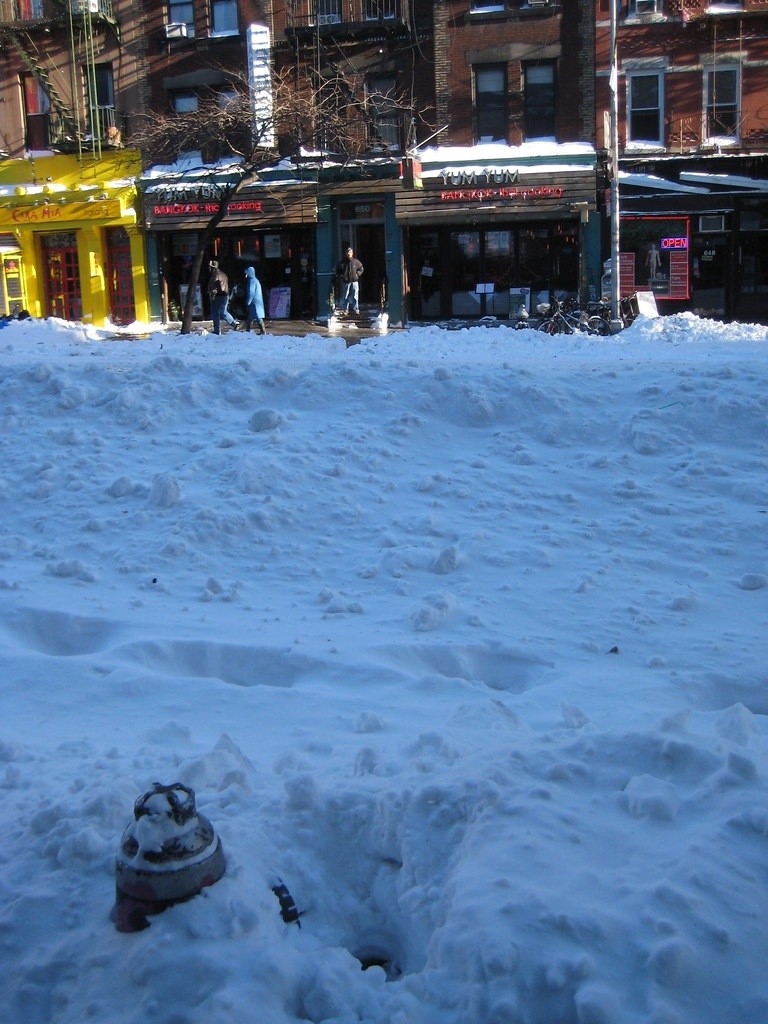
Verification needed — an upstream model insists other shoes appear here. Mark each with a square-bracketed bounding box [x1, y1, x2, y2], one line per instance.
[234, 324, 240, 330]
[257, 331, 265, 335]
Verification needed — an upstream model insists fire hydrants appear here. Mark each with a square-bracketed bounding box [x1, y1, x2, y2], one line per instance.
[112, 780, 300, 937]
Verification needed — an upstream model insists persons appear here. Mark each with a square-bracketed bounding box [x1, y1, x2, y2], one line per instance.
[335, 247, 364, 316]
[244, 267, 268, 335]
[645, 244, 662, 279]
[209, 260, 241, 336]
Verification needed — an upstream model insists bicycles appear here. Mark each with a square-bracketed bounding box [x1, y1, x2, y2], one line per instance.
[535, 290, 637, 338]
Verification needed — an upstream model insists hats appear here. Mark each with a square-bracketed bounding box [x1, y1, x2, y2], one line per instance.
[209, 260, 218, 268]
